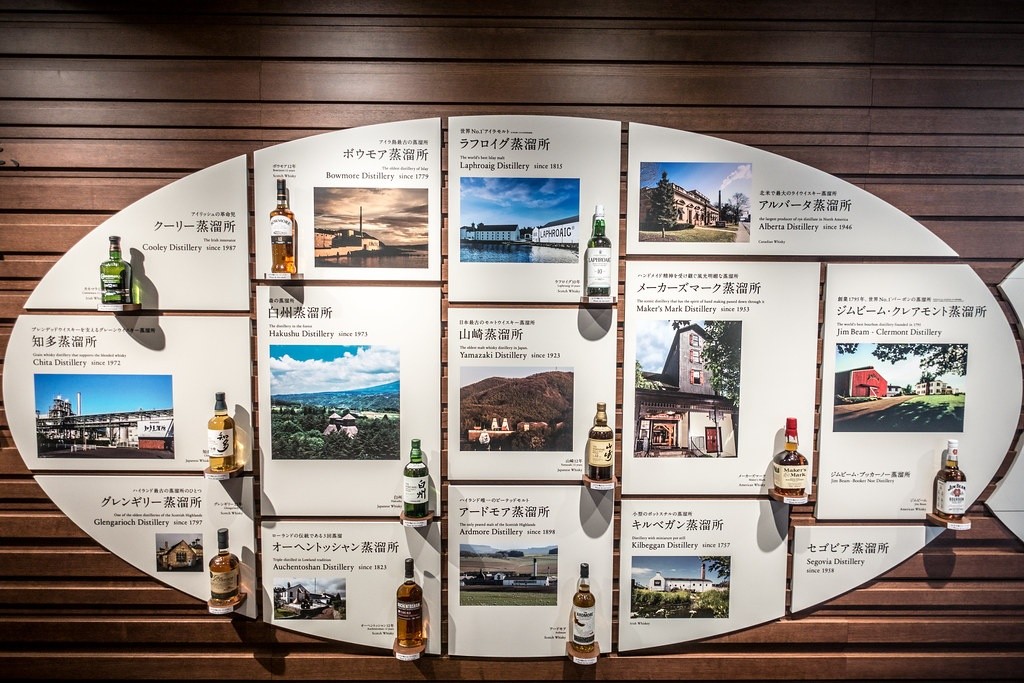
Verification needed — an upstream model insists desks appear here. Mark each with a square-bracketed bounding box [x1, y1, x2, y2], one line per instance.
[469, 430, 516, 451]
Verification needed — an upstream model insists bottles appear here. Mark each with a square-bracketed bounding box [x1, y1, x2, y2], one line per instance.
[403, 438, 429, 518]
[584, 206, 612, 297]
[772, 418, 808, 497]
[587, 402, 613, 481]
[207, 392, 236, 473]
[572, 562, 595, 652]
[98, 235, 134, 304]
[397, 558, 423, 648]
[208, 529, 241, 606]
[269, 178, 298, 274]
[935, 440, 967, 522]
